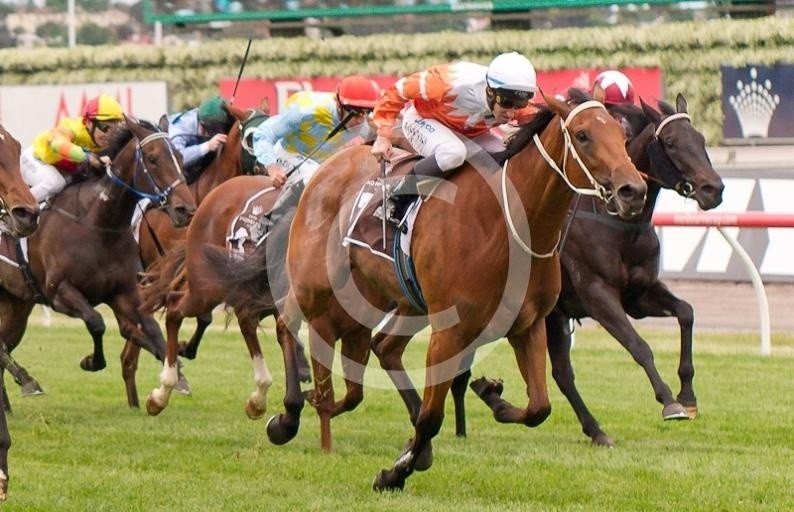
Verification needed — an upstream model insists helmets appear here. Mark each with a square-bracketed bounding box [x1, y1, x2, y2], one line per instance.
[486, 51, 537, 93]
[197, 97, 230, 131]
[592, 71, 634, 106]
[81, 95, 125, 126]
[336, 76, 381, 108]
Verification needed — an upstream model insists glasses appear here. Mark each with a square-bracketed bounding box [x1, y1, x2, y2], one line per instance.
[344, 108, 370, 118]
[493, 93, 528, 109]
[96, 123, 111, 133]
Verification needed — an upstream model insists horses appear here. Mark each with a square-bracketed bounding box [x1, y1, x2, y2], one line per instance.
[0, 80, 726, 501]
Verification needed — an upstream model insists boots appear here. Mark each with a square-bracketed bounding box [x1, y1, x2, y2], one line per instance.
[372, 167, 421, 235]
[255, 186, 297, 242]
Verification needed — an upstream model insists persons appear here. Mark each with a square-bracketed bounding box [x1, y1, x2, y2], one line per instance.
[20, 95, 124, 203]
[249, 76, 384, 245]
[554, 69, 635, 143]
[372, 50, 537, 235]
[150, 96, 232, 166]
